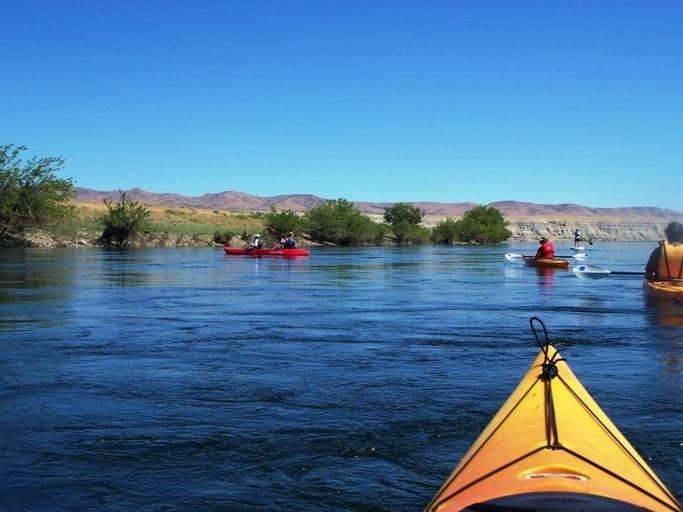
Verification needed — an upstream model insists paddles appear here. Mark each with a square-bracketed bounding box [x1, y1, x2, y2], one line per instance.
[505, 253, 589, 261]
[572, 265, 647, 275]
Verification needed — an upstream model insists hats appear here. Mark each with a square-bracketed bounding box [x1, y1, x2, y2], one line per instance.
[255, 234, 260, 237]
[538, 237, 548, 242]
[286, 232, 294, 236]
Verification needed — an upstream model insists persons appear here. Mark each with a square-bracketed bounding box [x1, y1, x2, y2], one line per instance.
[532, 235, 555, 260]
[643, 221, 682, 280]
[571, 228, 581, 247]
[283, 231, 296, 249]
[247, 233, 263, 249]
[270, 234, 284, 248]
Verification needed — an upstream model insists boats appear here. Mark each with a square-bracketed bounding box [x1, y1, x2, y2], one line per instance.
[422, 317, 683, 512]
[569, 247, 585, 250]
[524, 257, 571, 269]
[641, 276, 683, 303]
[223, 246, 310, 256]
